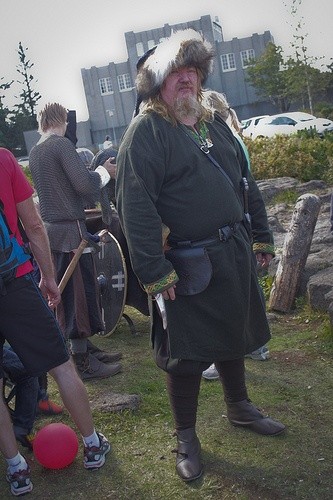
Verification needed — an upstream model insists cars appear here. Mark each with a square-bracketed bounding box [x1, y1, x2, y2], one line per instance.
[251, 112, 333, 142]
[241, 115, 272, 138]
[75, 146, 95, 168]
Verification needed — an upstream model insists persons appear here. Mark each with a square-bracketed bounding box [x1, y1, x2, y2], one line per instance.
[0, 147, 111, 496]
[3, 343, 63, 452]
[114, 28, 285, 481]
[202, 91, 269, 380]
[29, 102, 124, 381]
[103, 135, 113, 149]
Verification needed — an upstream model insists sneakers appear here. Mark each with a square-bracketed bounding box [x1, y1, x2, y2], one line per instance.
[83, 432, 109, 469]
[5, 464, 32, 495]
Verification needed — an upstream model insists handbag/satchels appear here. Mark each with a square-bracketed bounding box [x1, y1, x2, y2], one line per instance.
[163, 248, 212, 296]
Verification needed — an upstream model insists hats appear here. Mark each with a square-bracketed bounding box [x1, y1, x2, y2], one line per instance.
[134, 28, 214, 97]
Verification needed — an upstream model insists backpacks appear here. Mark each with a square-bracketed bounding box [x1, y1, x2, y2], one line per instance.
[0, 198, 33, 286]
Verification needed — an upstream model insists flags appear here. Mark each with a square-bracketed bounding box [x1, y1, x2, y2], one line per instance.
[33, 423, 79, 469]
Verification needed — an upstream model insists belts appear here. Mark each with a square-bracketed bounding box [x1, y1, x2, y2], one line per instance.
[168, 222, 241, 248]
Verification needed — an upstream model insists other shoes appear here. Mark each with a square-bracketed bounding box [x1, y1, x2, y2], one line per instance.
[69, 352, 121, 380]
[86, 342, 121, 363]
[36, 393, 61, 413]
[174, 428, 203, 481]
[202, 362, 219, 379]
[225, 400, 286, 436]
[244, 343, 269, 360]
